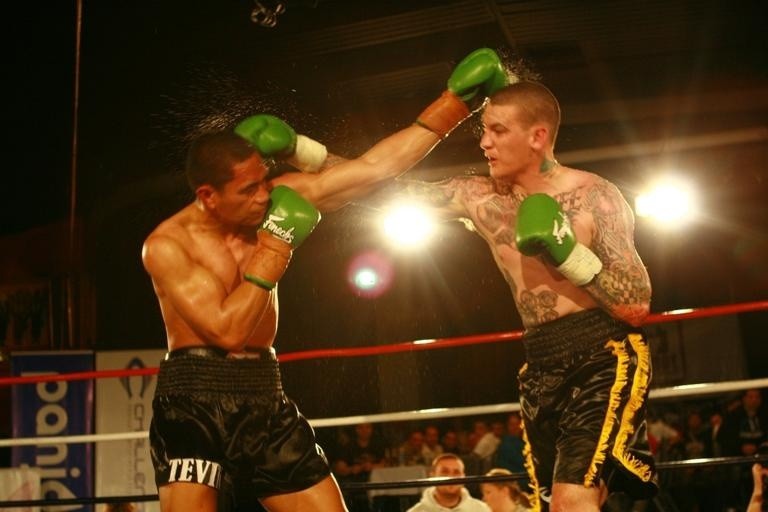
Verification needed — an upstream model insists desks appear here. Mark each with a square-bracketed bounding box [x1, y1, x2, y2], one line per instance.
[369, 465, 427, 512]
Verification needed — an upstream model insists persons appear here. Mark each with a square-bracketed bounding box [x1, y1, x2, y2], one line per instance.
[141, 47, 507, 512]
[325, 389, 767, 512]
[235, 81, 660, 512]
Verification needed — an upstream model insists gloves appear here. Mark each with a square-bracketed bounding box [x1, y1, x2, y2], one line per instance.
[516, 193, 603, 286]
[416, 47, 508, 139]
[243, 185, 322, 290]
[234, 115, 328, 174]
